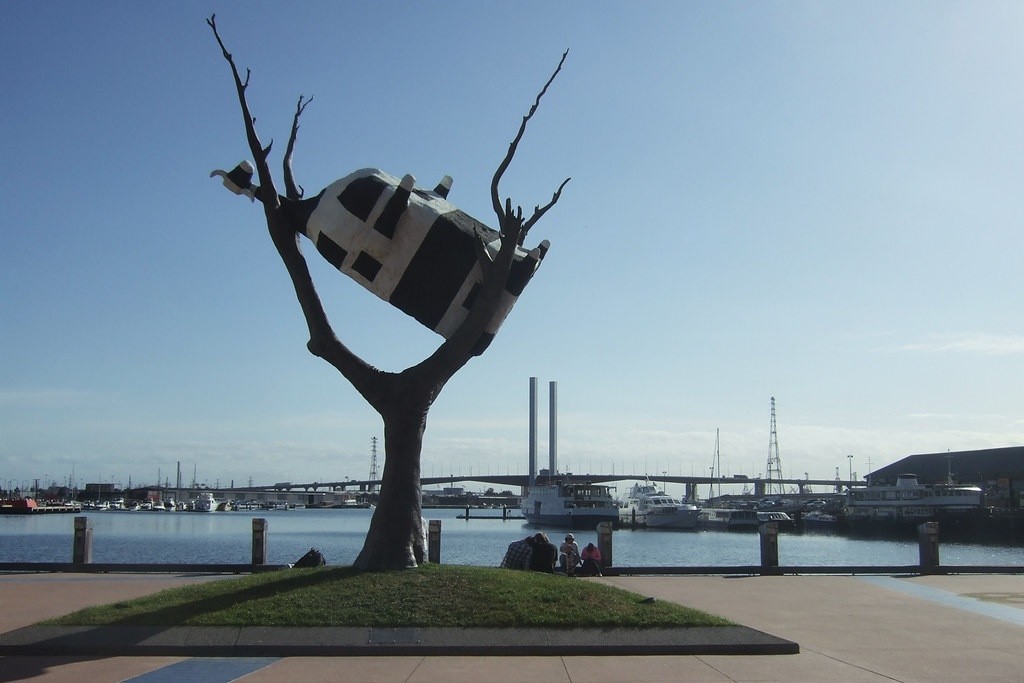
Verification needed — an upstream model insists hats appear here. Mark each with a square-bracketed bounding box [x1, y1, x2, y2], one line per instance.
[567, 533, 574, 539]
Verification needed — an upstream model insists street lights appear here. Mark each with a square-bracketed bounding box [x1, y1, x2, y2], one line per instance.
[847, 455, 853, 487]
[662, 471, 666, 493]
[8, 478, 17, 491]
[0, 478, 6, 489]
[709, 467, 714, 490]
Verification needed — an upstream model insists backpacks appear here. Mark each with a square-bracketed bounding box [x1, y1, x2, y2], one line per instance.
[581, 559, 600, 574]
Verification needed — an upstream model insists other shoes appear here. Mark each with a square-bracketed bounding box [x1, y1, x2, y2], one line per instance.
[598, 571, 603, 577]
[573, 570, 577, 576]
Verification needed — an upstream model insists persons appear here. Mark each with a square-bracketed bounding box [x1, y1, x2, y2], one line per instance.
[499, 533, 558, 573]
[560, 534, 580, 577]
[582, 543, 604, 577]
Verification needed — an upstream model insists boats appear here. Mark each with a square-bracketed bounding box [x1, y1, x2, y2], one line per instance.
[520, 469, 1024, 531]
[38, 493, 240, 512]
